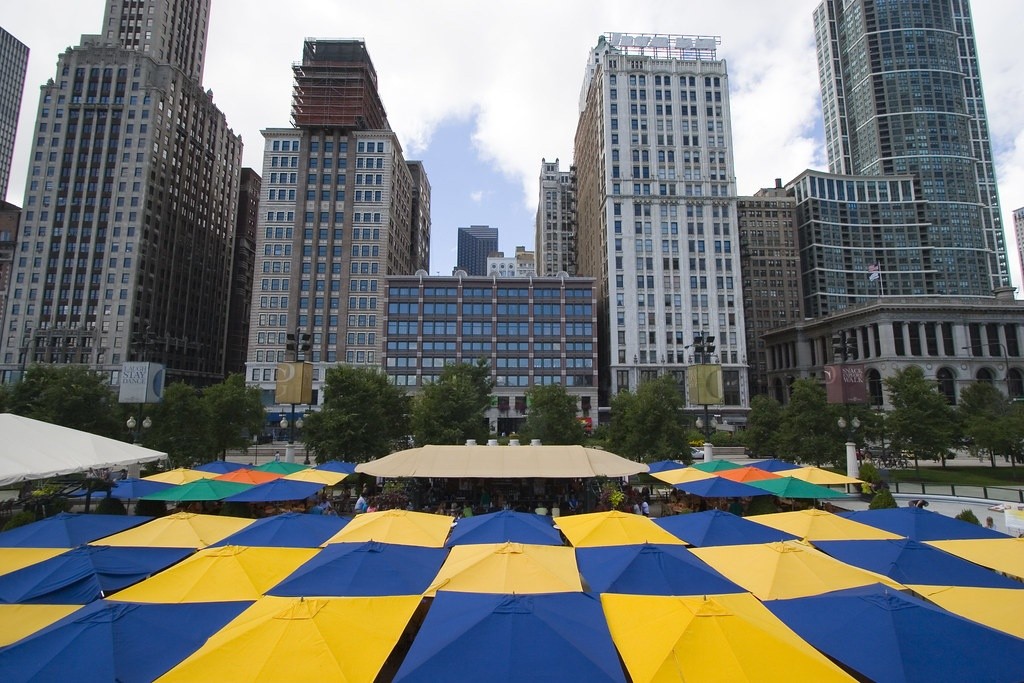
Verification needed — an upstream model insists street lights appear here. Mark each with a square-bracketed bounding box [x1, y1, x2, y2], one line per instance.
[116, 326, 166, 514]
[824, 329, 869, 492]
[685, 331, 725, 462]
[963, 342, 1011, 402]
[272, 326, 313, 466]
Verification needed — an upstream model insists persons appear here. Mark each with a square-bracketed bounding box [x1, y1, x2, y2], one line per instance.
[909, 499, 929, 510]
[310, 480, 840, 544]
[984, 516, 996, 531]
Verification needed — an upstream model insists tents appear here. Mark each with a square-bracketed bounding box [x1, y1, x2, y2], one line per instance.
[0, 413, 167, 484]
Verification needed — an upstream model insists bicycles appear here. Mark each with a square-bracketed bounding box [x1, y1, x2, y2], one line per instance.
[868, 457, 914, 469]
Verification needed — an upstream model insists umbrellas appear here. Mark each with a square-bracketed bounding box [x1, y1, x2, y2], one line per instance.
[646, 459, 867, 498]
[1, 509, 1024, 683]
[108, 459, 357, 503]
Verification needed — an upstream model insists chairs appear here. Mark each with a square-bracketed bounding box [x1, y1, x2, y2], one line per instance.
[0, 497, 16, 517]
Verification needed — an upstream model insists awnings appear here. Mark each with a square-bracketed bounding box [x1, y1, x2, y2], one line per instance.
[354, 444, 649, 478]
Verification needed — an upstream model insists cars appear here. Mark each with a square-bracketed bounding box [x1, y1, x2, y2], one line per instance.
[688, 447, 705, 459]
[388, 435, 417, 450]
[856, 445, 918, 461]
